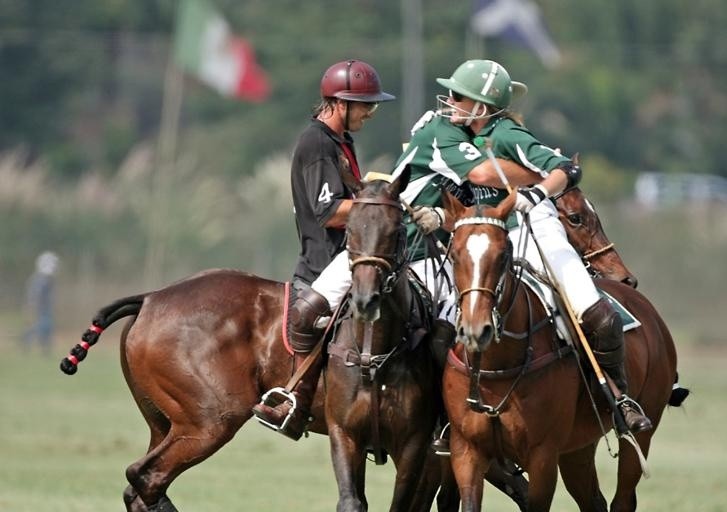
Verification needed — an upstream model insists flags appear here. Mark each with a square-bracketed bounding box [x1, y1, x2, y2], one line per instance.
[468, 0, 562, 71]
[165, 0, 271, 102]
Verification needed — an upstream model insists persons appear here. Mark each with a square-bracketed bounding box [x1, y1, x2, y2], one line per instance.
[14, 250, 61, 357]
[251, 80, 546, 441]
[286, 57, 396, 286]
[409, 59, 654, 437]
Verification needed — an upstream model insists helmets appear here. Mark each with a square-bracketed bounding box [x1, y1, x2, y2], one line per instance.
[435, 59, 514, 110]
[317, 58, 397, 103]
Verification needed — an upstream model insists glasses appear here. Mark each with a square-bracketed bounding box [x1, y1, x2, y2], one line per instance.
[447, 87, 467, 103]
[355, 100, 379, 113]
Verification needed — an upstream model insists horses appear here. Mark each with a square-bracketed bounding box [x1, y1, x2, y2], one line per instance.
[59, 148, 689, 511]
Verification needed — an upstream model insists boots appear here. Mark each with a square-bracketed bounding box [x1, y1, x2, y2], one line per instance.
[430, 425, 452, 452]
[253, 351, 324, 441]
[583, 298, 654, 434]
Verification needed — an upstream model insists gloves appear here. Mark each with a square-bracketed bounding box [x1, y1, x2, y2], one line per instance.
[409, 108, 435, 137]
[510, 183, 547, 215]
[407, 204, 446, 236]
